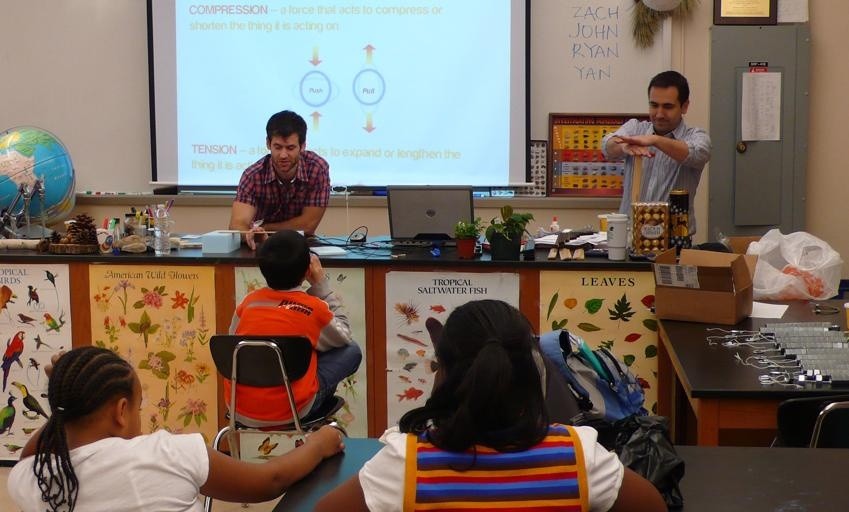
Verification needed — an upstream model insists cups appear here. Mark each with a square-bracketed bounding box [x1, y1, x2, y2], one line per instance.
[608, 215, 628, 261]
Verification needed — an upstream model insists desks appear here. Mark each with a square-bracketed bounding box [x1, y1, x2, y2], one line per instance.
[653, 296, 849, 448]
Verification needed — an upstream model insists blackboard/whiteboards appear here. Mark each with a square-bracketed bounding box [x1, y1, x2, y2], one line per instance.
[0, 1, 686, 210]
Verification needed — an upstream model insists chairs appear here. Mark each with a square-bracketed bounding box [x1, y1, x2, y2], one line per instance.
[770, 394, 849, 450]
[200, 334, 351, 511]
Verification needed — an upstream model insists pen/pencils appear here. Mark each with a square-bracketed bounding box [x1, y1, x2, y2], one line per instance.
[87, 190, 118, 196]
[147, 198, 174, 217]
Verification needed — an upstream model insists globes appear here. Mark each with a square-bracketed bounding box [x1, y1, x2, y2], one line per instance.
[0, 124, 75, 238]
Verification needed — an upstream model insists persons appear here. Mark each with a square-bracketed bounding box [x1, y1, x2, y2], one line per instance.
[601, 71, 713, 248]
[224, 229, 362, 428]
[229, 109, 331, 251]
[7, 345, 346, 512]
[311, 299, 669, 512]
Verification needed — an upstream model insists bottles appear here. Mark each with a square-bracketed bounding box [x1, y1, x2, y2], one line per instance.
[122, 214, 140, 238]
[550, 216, 560, 235]
[156, 204, 170, 255]
[670, 190, 689, 254]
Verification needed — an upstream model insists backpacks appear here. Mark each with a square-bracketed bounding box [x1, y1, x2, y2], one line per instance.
[536, 329, 649, 455]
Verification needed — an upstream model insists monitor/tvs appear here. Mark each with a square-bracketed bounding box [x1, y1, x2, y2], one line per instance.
[388, 183, 474, 249]
